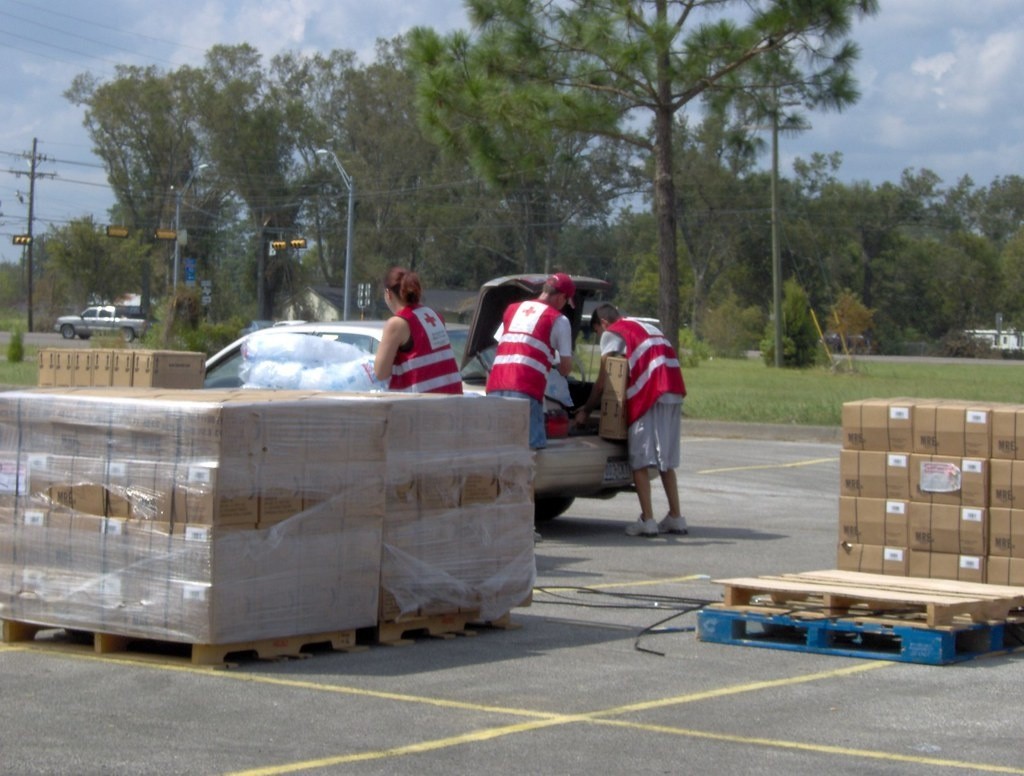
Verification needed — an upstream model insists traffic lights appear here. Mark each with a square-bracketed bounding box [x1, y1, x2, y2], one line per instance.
[272, 241, 286, 249]
[290, 239, 307, 248]
[13, 235, 32, 244]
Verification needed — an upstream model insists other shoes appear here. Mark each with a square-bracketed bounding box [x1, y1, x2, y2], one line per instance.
[533, 528, 542, 542]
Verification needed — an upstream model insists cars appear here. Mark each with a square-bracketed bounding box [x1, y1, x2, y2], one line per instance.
[201, 274, 661, 521]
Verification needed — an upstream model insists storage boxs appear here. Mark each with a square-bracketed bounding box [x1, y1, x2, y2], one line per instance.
[597, 356, 631, 439]
[836, 397, 1023, 587]
[0, 348, 538, 648]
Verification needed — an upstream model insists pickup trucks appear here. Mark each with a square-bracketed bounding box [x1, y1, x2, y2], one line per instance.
[53, 305, 145, 342]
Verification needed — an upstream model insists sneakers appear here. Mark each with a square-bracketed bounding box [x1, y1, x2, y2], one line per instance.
[658, 513, 688, 533]
[625, 513, 659, 536]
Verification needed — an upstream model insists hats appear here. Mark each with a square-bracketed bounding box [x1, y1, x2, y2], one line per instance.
[546, 273, 576, 309]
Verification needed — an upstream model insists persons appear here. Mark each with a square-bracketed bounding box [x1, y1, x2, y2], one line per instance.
[482, 274, 575, 544]
[373, 265, 465, 395]
[574, 304, 690, 537]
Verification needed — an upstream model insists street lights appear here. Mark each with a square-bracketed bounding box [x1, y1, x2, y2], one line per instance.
[173, 164, 208, 293]
[316, 148, 355, 322]
